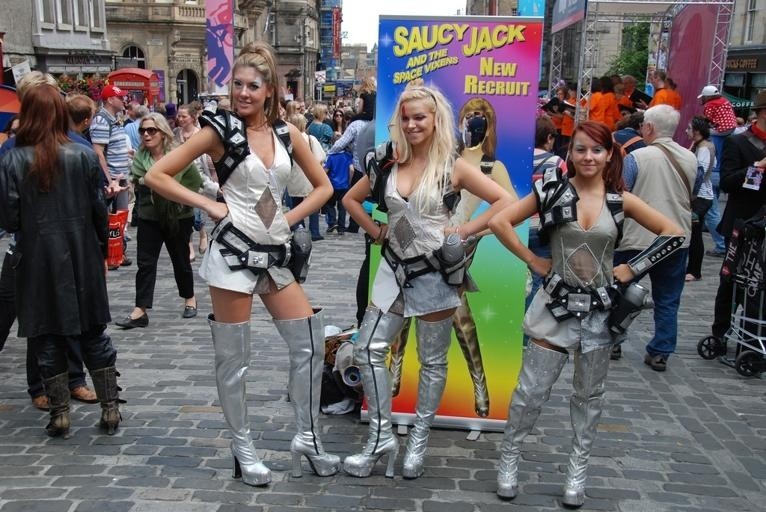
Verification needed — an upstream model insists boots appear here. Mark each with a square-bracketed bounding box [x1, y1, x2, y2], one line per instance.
[390, 316, 412, 397]
[453, 293, 489, 417]
[89, 366, 124, 434]
[44, 371, 70, 439]
[272, 306, 341, 478]
[343, 307, 400, 477]
[498, 337, 569, 498]
[402, 314, 455, 477]
[563, 344, 614, 506]
[208, 313, 270, 486]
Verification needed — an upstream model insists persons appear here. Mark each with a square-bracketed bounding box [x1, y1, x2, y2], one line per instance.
[145, 42, 342, 483]
[488, 122, 685, 502]
[2, 42, 765, 438]
[341, 83, 514, 478]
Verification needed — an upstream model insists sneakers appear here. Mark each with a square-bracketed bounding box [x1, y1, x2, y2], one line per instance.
[311, 222, 357, 241]
[199, 232, 208, 255]
[611, 345, 621, 359]
[645, 353, 666, 371]
[190, 256, 196, 262]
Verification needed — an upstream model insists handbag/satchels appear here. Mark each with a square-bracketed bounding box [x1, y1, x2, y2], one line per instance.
[103, 196, 129, 267]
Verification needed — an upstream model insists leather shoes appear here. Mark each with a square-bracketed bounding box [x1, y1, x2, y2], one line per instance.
[70, 386, 99, 403]
[183, 301, 197, 318]
[31, 396, 50, 410]
[115, 313, 148, 328]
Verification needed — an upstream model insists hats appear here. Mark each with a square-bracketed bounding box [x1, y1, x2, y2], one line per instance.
[750, 89, 766, 109]
[101, 86, 129, 99]
[697, 85, 720, 100]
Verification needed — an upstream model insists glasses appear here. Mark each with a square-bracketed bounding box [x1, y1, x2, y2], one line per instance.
[138, 127, 161, 135]
